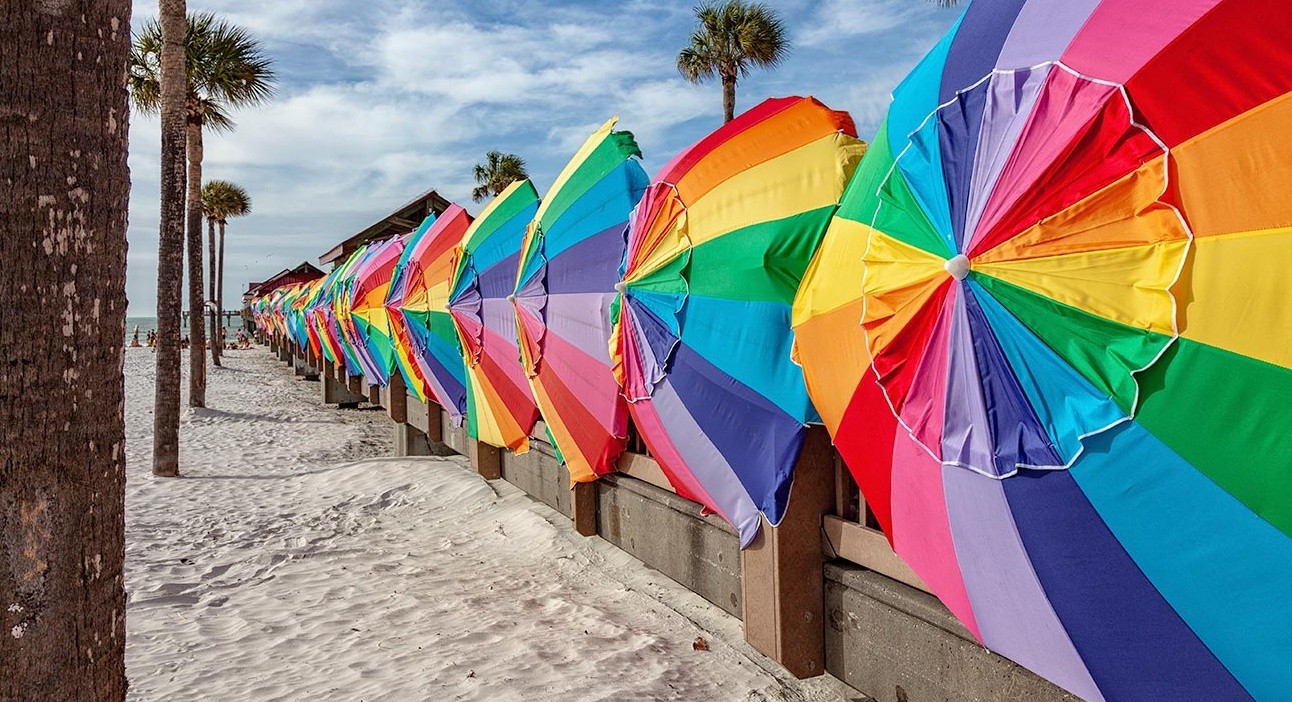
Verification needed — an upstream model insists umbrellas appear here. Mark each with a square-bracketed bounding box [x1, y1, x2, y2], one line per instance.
[506, 119, 652, 492]
[790, 0, 1292, 702]
[445, 176, 543, 456]
[381, 211, 438, 404]
[608, 96, 873, 553]
[250, 239, 383, 376]
[396, 202, 471, 428]
[346, 234, 406, 389]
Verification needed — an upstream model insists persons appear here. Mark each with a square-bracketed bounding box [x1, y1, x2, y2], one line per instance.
[222, 327, 227, 349]
[235, 328, 250, 347]
[132, 329, 158, 352]
[181, 335, 189, 348]
